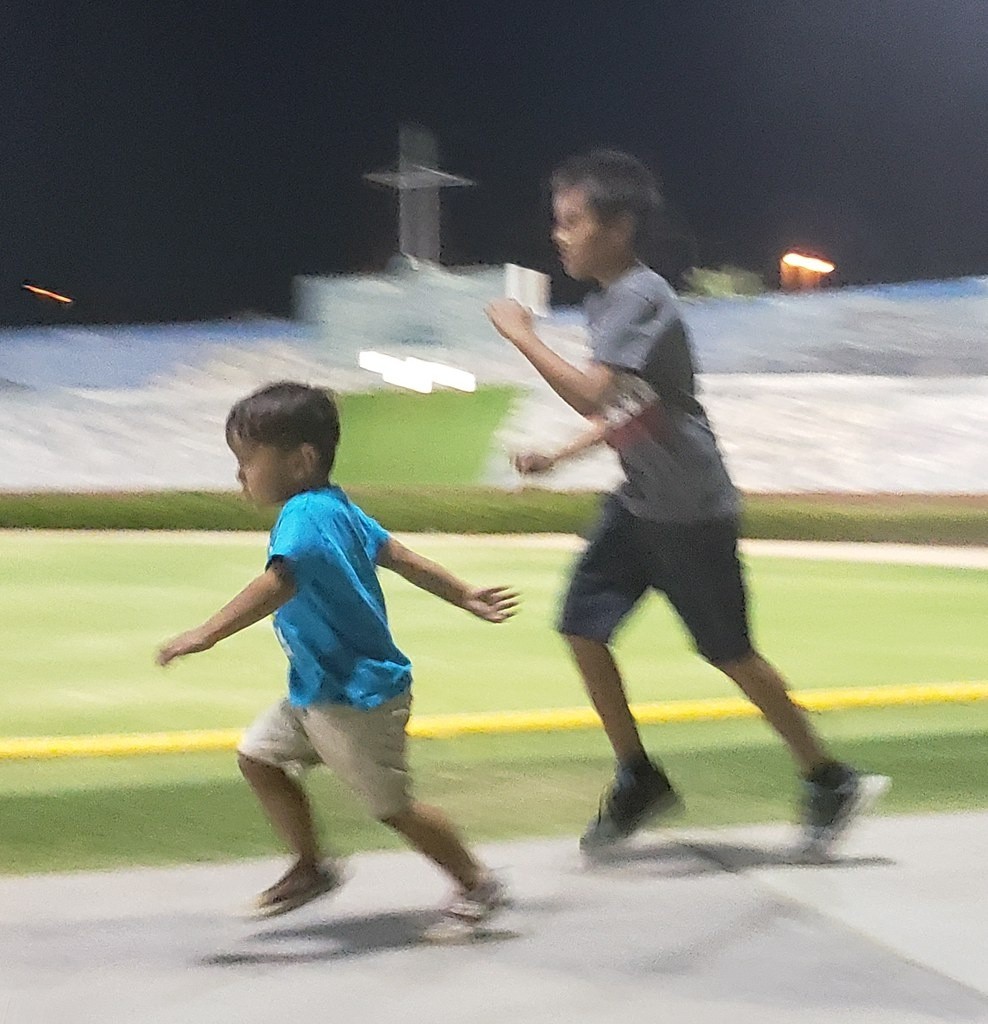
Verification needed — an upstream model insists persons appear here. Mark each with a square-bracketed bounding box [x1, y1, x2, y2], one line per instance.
[154, 383, 525, 938]
[487, 148, 864, 865]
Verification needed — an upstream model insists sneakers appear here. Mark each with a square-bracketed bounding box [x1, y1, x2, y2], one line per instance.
[791, 762, 875, 863]
[419, 877, 516, 946]
[579, 767, 680, 855]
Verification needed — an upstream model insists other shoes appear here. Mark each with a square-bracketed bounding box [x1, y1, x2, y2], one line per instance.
[251, 858, 348, 921]
